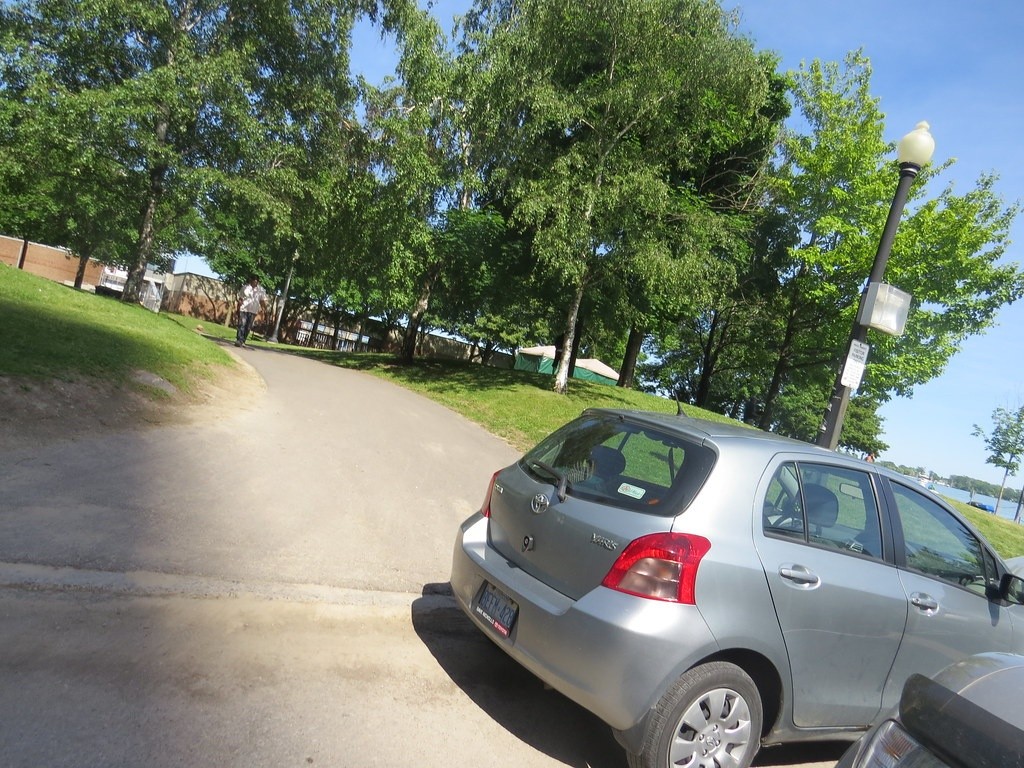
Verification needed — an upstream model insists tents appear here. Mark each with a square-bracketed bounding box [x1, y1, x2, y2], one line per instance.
[514, 346, 620, 385]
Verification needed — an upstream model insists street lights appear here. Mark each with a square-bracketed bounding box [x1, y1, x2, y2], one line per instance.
[803, 120, 935, 484]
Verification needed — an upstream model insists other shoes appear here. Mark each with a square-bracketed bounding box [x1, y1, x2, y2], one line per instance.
[235, 341, 245, 346]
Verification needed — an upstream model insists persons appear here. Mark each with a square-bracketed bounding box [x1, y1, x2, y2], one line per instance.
[864, 452, 874, 463]
[235, 275, 267, 347]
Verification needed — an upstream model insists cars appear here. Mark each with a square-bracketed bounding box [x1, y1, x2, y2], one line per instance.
[451, 407, 1024, 768]
[832, 651, 1024, 768]
[968, 502, 994, 514]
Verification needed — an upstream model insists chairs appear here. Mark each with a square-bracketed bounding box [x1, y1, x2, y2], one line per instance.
[590, 445, 627, 481]
[773, 483, 839, 537]
